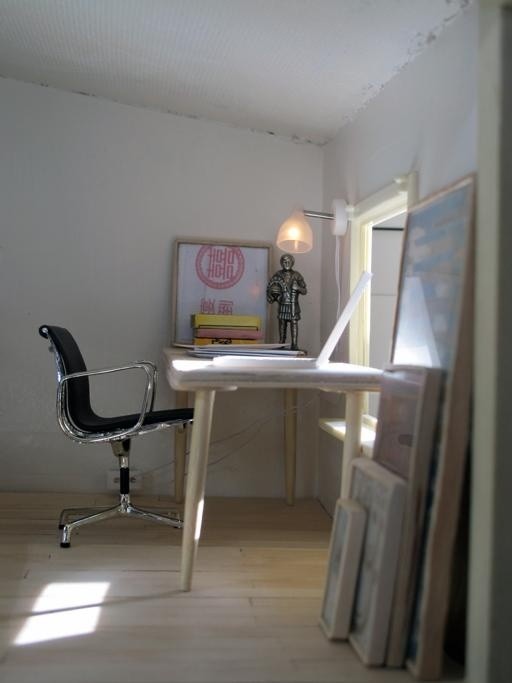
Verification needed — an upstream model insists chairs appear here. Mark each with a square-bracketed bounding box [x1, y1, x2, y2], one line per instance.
[39, 324, 195, 549]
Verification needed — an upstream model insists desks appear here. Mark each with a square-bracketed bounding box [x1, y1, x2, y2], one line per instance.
[162, 347, 384, 592]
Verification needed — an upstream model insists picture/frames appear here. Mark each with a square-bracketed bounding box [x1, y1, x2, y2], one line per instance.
[345, 457, 407, 667]
[389, 173, 478, 682]
[169, 236, 274, 350]
[316, 498, 368, 640]
[373, 366, 445, 670]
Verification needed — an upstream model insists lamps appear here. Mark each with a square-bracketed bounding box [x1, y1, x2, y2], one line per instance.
[276, 198, 348, 253]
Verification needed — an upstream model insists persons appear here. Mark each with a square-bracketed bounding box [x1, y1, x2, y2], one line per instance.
[267, 254, 308, 351]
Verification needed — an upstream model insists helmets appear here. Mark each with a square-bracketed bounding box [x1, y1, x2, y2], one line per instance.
[270, 282, 283, 302]
